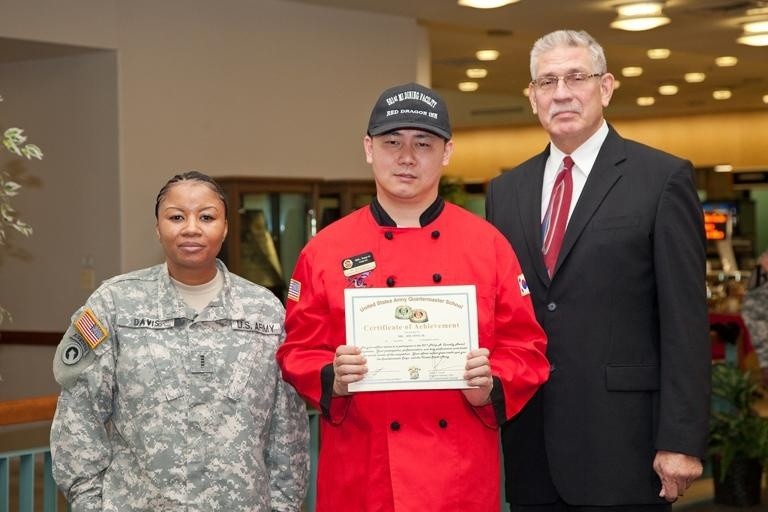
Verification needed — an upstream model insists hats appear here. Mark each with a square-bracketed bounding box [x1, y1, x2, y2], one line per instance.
[368, 83, 452, 140]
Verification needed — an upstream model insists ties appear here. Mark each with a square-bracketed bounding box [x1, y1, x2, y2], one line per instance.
[541, 156, 575, 280]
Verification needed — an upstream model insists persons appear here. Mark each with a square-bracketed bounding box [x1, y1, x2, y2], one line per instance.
[484, 27, 712, 511]
[49, 171, 312, 511]
[272, 82, 553, 512]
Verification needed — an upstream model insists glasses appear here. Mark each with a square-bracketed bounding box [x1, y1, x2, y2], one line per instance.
[532, 72, 605, 88]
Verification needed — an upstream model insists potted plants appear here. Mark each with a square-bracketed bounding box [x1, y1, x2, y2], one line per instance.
[704, 363, 768, 507]
